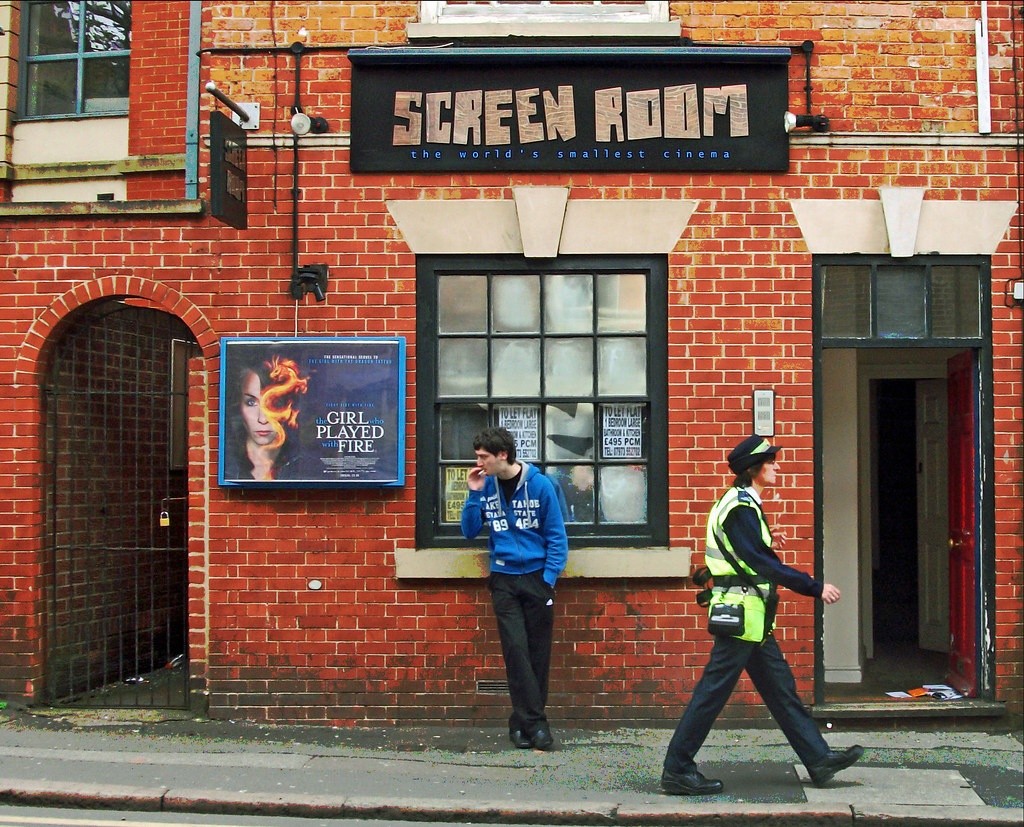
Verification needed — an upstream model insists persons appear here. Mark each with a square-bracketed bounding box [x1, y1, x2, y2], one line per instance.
[660, 434, 865, 796]
[461, 427, 569, 750]
[226, 351, 303, 482]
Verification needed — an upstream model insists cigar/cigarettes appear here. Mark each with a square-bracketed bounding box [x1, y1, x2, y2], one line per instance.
[478, 468, 484, 475]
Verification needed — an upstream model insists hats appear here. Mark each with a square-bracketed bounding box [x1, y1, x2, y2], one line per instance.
[727, 434, 782, 474]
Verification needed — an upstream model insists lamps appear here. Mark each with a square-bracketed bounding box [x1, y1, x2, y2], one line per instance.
[786, 112, 830, 133]
[291, 114, 329, 136]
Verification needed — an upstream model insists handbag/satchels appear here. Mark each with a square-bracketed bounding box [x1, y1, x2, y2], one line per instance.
[707, 603, 745, 636]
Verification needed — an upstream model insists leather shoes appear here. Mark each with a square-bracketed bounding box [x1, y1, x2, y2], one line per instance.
[531, 728, 552, 750]
[509, 728, 534, 748]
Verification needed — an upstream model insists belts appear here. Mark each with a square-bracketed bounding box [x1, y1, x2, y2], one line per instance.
[713, 575, 769, 587]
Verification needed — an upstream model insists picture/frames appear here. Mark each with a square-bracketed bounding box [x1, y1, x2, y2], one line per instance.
[220, 337, 407, 487]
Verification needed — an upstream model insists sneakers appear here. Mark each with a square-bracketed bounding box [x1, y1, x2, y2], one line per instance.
[807, 744, 864, 785]
[661, 768, 723, 796]
